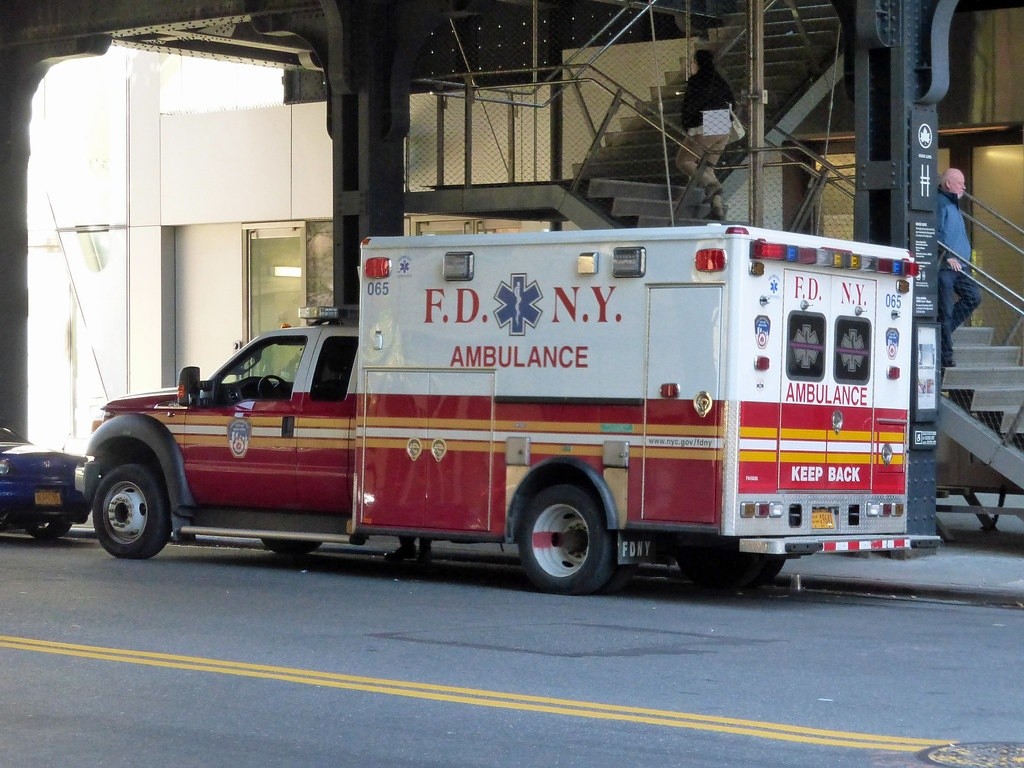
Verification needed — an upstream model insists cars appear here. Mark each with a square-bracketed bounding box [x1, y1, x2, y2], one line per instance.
[1, 425, 91, 542]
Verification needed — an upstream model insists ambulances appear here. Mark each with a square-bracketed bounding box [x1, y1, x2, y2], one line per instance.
[75, 222, 919, 595]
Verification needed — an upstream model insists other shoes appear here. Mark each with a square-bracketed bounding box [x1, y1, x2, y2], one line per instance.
[383, 545, 416, 560]
[417, 551, 432, 563]
[703, 208, 723, 221]
[942, 360, 956, 367]
[701, 182, 723, 204]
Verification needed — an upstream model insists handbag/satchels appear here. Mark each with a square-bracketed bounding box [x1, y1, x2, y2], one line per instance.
[728, 102, 745, 144]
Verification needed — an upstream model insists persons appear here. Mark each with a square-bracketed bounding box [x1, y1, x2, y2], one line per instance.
[384, 534, 432, 571]
[936, 168, 982, 368]
[675, 50, 735, 219]
